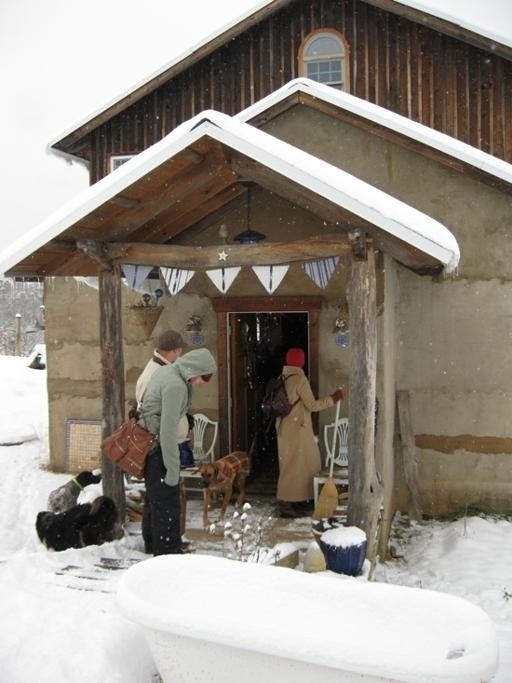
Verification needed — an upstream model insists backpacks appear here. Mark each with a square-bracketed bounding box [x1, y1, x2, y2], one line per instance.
[262, 372, 299, 417]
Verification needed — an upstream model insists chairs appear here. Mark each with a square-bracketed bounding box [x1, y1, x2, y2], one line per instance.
[178, 412, 220, 503]
[313, 418, 352, 514]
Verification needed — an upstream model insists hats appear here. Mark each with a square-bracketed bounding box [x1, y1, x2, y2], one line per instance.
[285, 347, 306, 367]
[158, 330, 189, 350]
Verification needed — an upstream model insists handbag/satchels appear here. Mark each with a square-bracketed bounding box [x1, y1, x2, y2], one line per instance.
[99, 416, 157, 479]
[128, 402, 142, 422]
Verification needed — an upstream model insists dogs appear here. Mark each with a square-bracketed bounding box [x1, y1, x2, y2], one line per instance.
[37, 496, 117, 552]
[199, 451, 250, 528]
[47, 471, 100, 515]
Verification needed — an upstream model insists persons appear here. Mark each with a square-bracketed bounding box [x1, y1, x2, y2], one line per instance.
[134, 328, 194, 549]
[269, 347, 346, 516]
[128, 345, 218, 555]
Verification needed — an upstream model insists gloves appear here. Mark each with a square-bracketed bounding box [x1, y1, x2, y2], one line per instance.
[160, 480, 179, 500]
[329, 388, 346, 404]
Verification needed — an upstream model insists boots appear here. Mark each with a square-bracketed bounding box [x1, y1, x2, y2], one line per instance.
[278, 501, 314, 517]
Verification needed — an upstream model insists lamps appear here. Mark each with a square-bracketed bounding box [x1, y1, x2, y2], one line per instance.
[234, 182, 266, 242]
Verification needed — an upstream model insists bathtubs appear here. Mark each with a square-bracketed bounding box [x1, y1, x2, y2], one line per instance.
[118, 554, 497, 683]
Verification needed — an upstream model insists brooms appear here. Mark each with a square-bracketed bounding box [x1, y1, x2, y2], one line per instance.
[313, 387, 341, 520]
